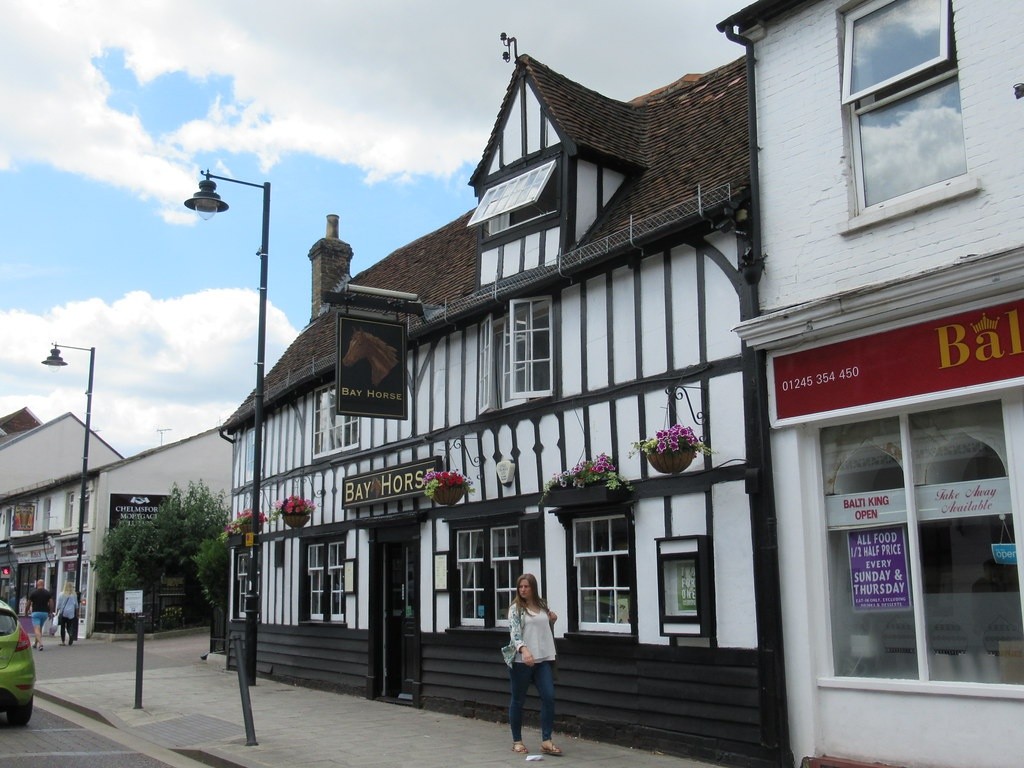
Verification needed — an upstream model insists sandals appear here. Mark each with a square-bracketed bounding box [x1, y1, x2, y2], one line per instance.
[540, 742, 562, 754]
[512, 743, 528, 753]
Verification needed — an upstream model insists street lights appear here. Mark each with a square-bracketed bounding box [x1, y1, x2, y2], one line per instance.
[183, 168, 272, 686]
[40, 342, 95, 641]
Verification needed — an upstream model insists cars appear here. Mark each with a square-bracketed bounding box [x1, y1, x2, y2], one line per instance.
[0, 598, 36, 727]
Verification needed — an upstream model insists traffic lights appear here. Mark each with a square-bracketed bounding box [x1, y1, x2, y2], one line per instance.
[3, 567, 10, 574]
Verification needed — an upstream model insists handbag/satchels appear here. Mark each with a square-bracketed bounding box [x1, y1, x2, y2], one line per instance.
[58, 613, 63, 625]
[552, 655, 560, 681]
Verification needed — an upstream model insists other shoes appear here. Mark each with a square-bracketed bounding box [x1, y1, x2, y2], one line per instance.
[32, 644, 37, 648]
[39, 645, 44, 651]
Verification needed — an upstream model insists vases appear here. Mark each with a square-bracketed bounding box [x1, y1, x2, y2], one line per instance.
[227, 534, 244, 546]
[283, 514, 311, 528]
[431, 485, 464, 505]
[549, 485, 626, 507]
[239, 523, 257, 535]
[646, 448, 696, 474]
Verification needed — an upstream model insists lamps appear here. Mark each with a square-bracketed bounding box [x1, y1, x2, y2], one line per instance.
[740, 247, 762, 285]
[1013, 83, 1024, 99]
[500, 33, 521, 63]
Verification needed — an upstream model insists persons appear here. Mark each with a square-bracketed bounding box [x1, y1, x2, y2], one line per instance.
[501, 574, 563, 755]
[25, 579, 53, 651]
[54, 582, 78, 647]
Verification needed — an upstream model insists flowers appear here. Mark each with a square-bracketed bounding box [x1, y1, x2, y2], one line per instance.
[538, 451, 639, 506]
[232, 508, 270, 528]
[217, 521, 242, 542]
[421, 470, 475, 499]
[628, 423, 720, 459]
[273, 496, 315, 516]
